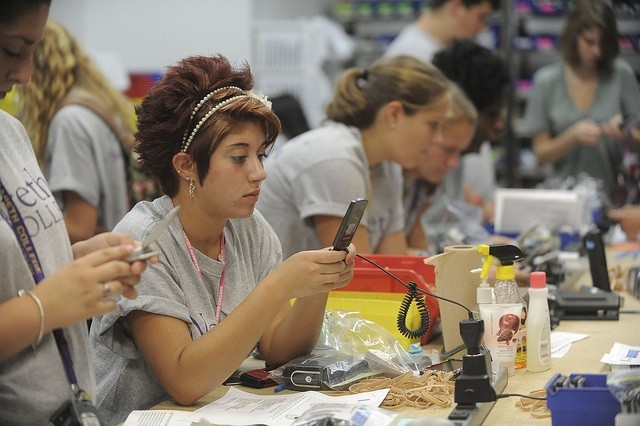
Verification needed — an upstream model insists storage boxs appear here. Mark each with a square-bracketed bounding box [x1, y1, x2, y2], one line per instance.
[334, 254, 438, 345]
[290, 288, 425, 355]
[545, 372, 619, 426]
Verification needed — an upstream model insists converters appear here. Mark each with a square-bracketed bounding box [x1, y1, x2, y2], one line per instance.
[454, 376, 498, 405]
[457, 318, 493, 372]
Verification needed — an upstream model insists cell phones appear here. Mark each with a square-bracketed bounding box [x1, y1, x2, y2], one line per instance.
[123, 205, 181, 262]
[328, 198, 369, 253]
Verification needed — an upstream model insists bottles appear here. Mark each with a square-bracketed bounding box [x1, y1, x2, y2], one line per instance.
[524, 271, 552, 374]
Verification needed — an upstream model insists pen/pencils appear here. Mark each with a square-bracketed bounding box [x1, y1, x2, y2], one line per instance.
[275, 384, 285, 392]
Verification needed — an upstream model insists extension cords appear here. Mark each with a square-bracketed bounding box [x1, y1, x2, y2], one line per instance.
[447, 367, 508, 426]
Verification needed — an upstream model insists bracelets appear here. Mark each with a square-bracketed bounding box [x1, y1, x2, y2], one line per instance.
[18, 289, 45, 355]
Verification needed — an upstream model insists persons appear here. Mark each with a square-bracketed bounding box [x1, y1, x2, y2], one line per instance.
[16, 22, 135, 245]
[402, 81, 477, 251]
[526, 1, 640, 203]
[1, 1, 159, 426]
[385, 1, 500, 63]
[255, 55, 451, 256]
[88, 54, 355, 426]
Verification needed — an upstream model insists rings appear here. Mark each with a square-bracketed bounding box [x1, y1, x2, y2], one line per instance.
[103, 283, 111, 297]
